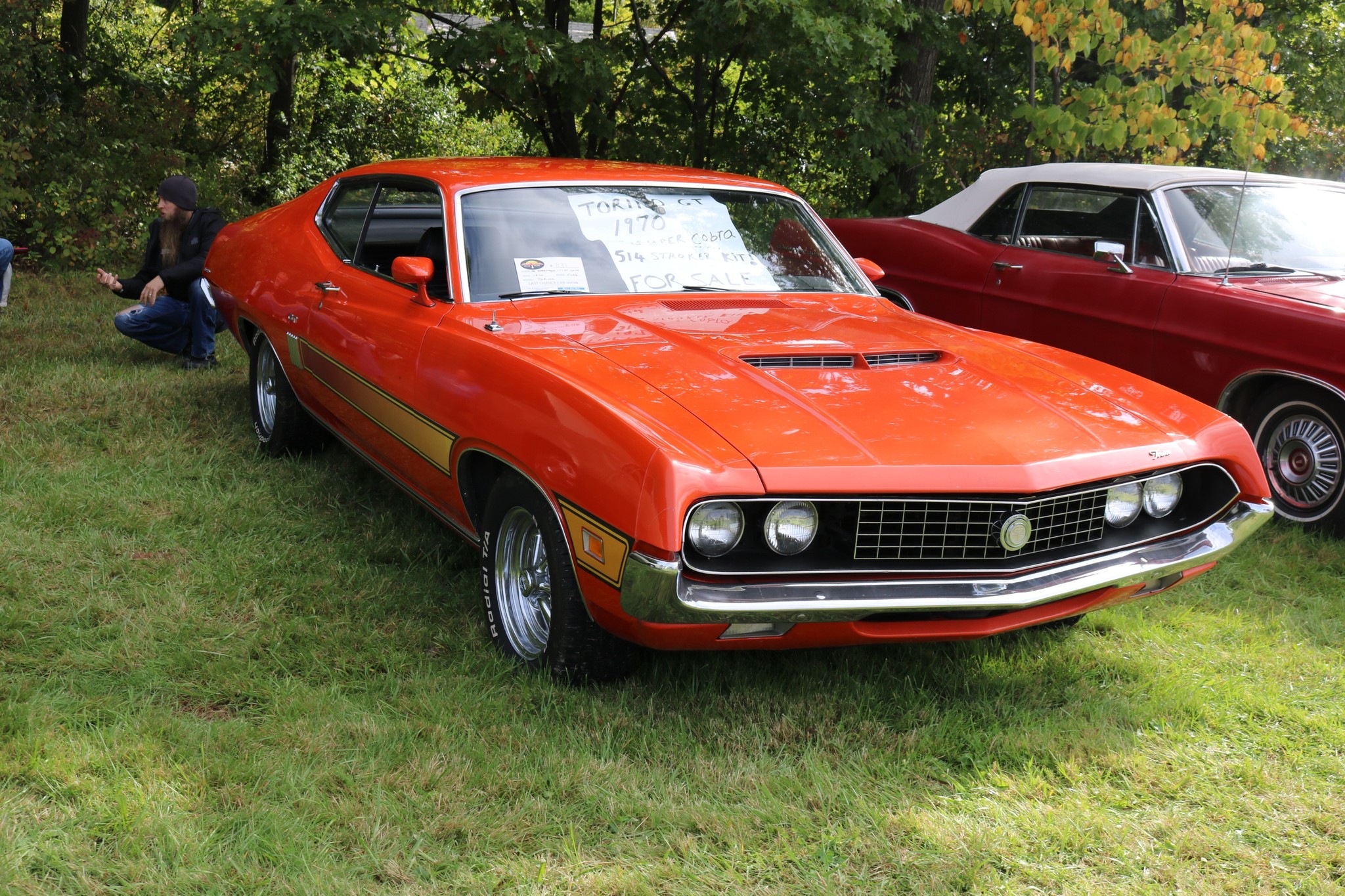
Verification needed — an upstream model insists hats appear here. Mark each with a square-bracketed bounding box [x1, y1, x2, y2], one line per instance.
[156, 174, 198, 211]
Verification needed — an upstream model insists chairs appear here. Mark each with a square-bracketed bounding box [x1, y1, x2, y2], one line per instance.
[1192, 257, 1253, 273]
[417, 226, 508, 302]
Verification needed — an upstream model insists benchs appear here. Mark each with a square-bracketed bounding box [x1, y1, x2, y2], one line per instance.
[996, 235, 1106, 255]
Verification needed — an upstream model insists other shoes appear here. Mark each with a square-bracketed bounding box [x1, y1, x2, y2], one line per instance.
[173, 351, 221, 371]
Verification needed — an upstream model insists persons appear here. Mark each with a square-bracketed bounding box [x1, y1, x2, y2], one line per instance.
[97, 177, 232, 368]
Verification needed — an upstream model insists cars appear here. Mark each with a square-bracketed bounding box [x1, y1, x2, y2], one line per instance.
[199, 158, 1273, 692]
[771, 163, 1345, 537]
